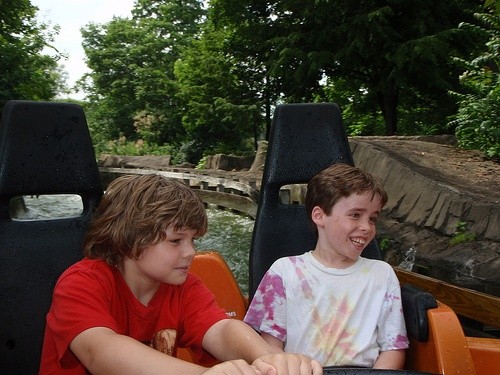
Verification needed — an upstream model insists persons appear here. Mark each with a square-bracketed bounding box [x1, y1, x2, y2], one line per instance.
[38, 173, 323, 375]
[243, 166, 410, 370]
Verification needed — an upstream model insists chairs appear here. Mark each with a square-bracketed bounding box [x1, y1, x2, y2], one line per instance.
[0, 101, 105, 375]
[248, 104, 384, 306]
